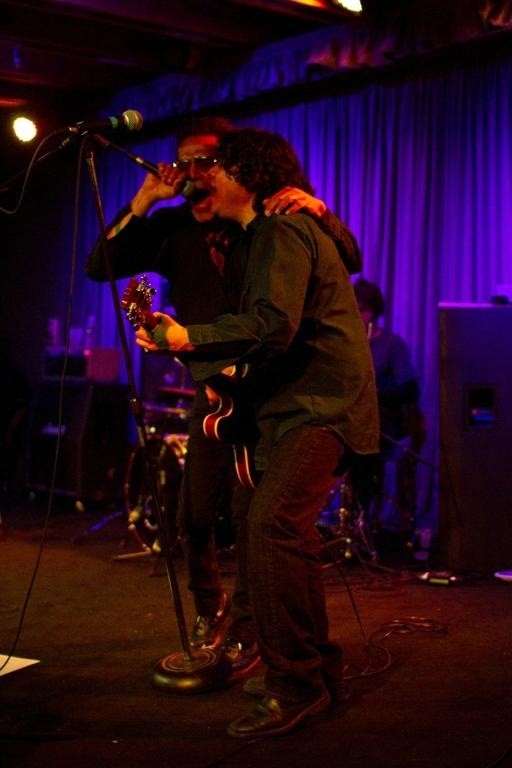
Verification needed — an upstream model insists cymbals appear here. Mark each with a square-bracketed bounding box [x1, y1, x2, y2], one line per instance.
[158, 388, 198, 397]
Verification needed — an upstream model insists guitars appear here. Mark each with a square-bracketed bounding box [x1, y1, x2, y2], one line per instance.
[119, 273, 267, 499]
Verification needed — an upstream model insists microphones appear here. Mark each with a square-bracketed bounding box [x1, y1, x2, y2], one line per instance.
[124, 152, 196, 198]
[69, 109, 143, 132]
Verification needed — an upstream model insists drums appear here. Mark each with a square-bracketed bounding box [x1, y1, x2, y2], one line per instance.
[124, 434, 189, 555]
[140, 407, 188, 434]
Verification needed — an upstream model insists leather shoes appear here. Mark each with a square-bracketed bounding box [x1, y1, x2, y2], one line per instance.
[191, 590, 230, 643]
[226, 686, 331, 738]
[242, 675, 266, 697]
[221, 635, 257, 669]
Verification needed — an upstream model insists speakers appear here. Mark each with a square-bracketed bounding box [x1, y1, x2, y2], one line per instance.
[22, 376, 131, 500]
[434, 306, 512, 574]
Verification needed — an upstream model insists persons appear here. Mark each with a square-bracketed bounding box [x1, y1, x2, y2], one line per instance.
[83, 111, 364, 689]
[335, 271, 426, 570]
[132, 122, 387, 741]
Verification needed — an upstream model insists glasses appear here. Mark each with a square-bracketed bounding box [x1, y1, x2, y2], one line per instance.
[177, 155, 218, 171]
